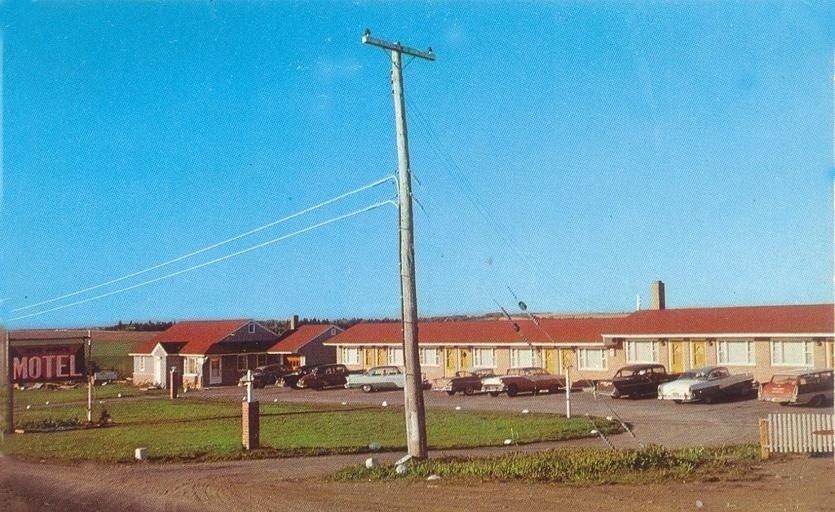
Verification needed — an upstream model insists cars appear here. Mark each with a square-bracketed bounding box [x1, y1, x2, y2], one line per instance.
[344, 366, 427, 392]
[239, 363, 286, 388]
[275, 364, 322, 388]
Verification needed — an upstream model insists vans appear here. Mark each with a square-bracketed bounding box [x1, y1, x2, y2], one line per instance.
[761, 368, 834, 406]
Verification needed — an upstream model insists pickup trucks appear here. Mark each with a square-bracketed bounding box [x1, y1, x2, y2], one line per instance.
[432, 368, 495, 395]
[657, 365, 754, 403]
[297, 363, 366, 390]
[481, 367, 565, 397]
[613, 363, 679, 398]
